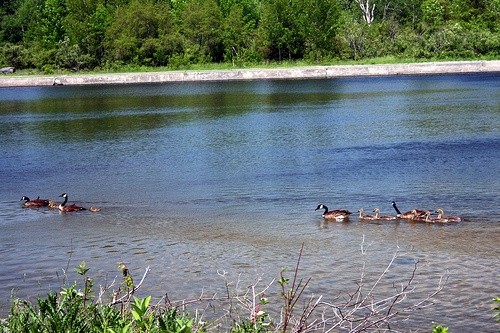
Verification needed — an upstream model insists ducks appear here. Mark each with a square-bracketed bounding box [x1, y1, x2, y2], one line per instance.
[435, 208, 462, 223]
[392, 201, 415, 218]
[58, 193, 88, 211]
[424, 210, 447, 223]
[372, 208, 396, 220]
[315, 204, 352, 218]
[357, 208, 376, 220]
[412, 208, 426, 220]
[89, 205, 102, 212]
[20, 196, 49, 206]
[48, 200, 58, 208]
